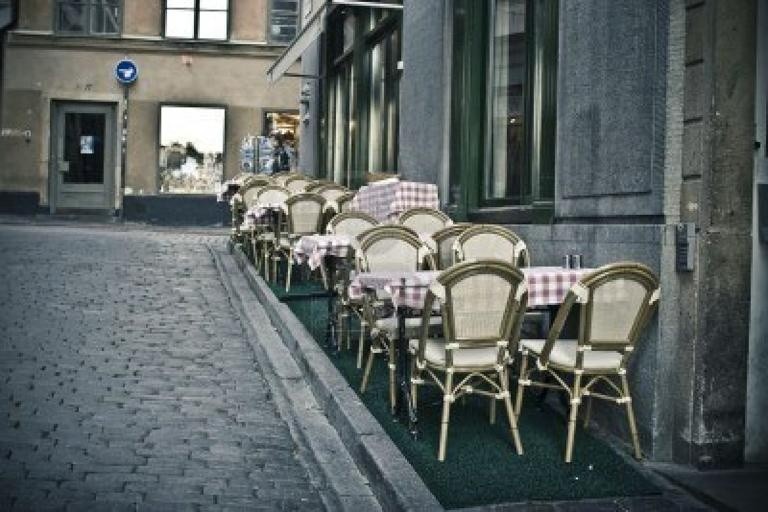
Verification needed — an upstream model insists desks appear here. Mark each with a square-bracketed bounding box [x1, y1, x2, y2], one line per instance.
[352, 265, 598, 426]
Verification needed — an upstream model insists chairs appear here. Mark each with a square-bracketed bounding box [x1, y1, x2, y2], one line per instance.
[515, 259, 660, 462]
[404, 257, 524, 463]
[230, 168, 531, 370]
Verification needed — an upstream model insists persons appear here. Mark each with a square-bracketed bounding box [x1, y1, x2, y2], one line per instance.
[265, 131, 290, 234]
